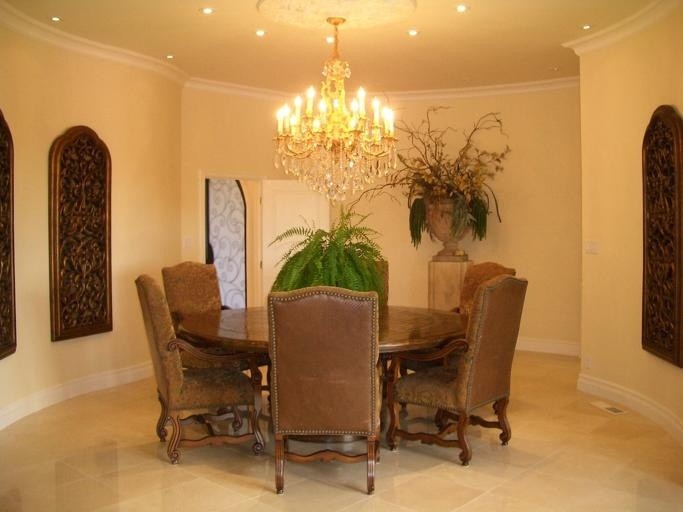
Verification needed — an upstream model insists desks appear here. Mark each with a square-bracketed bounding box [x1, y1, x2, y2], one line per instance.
[180, 303, 468, 444]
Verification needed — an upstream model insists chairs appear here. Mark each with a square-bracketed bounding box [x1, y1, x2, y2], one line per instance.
[160, 261, 267, 371]
[265, 285, 383, 494]
[375, 260, 389, 302]
[399, 261, 516, 407]
[133, 272, 266, 466]
[386, 273, 529, 467]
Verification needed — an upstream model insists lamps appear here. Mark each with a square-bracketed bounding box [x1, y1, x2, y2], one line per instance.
[272, 15, 399, 204]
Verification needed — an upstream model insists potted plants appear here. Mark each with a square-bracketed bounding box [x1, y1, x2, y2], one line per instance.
[397, 107, 517, 261]
